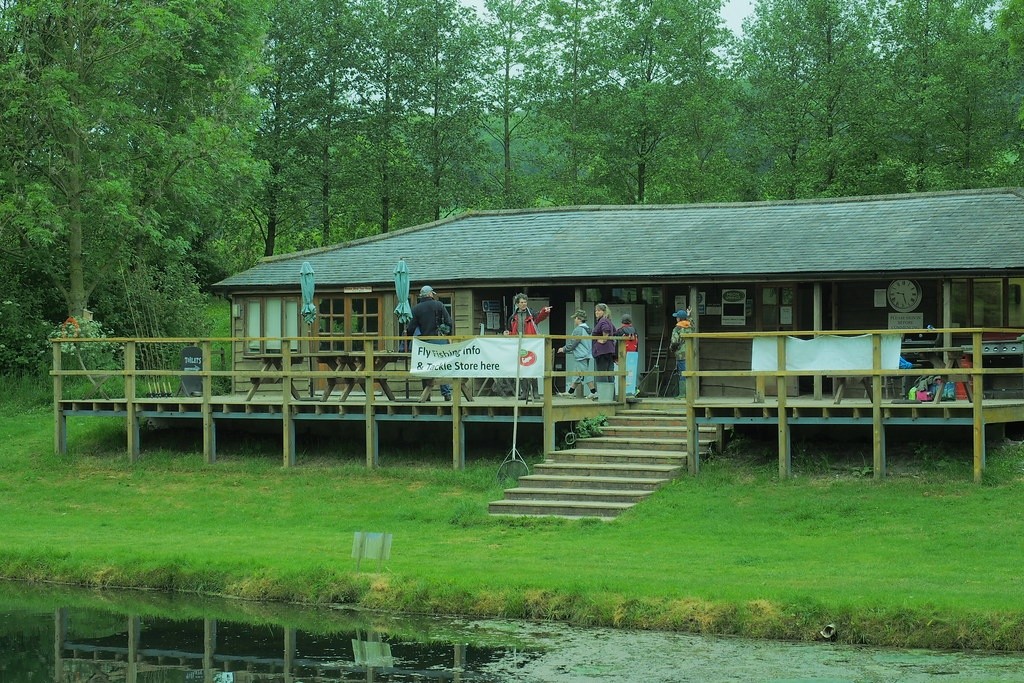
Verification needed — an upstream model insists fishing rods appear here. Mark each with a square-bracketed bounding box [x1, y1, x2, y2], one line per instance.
[119, 257, 173, 399]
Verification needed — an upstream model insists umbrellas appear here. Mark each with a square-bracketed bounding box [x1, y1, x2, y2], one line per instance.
[394, 257, 413, 398]
[299, 262, 317, 397]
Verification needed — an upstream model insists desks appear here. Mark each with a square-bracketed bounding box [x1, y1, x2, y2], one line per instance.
[243, 351, 475, 402]
[833, 345, 974, 405]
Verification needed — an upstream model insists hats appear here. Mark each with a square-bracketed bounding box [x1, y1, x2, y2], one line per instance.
[421, 286, 436, 294]
[621, 314, 632, 324]
[571, 310, 587, 320]
[672, 310, 687, 319]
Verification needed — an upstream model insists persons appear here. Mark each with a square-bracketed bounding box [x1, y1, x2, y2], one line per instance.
[616, 314, 638, 352]
[591, 303, 618, 400]
[557, 310, 598, 399]
[403, 286, 453, 401]
[669, 308, 695, 398]
[502, 293, 553, 400]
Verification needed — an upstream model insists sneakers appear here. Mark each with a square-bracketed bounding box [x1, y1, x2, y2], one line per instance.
[584, 392, 598, 399]
[559, 392, 574, 399]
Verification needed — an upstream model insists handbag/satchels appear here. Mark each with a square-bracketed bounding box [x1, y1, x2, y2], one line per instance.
[931, 382, 955, 402]
[436, 324, 450, 336]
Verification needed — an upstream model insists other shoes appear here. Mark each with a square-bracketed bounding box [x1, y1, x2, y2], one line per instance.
[445, 395, 451, 401]
[426, 395, 430, 401]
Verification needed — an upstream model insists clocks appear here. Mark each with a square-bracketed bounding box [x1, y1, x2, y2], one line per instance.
[887, 279, 922, 313]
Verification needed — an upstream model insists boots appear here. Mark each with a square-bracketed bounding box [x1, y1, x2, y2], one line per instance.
[518, 378, 528, 400]
[532, 378, 540, 399]
[674, 380, 686, 399]
